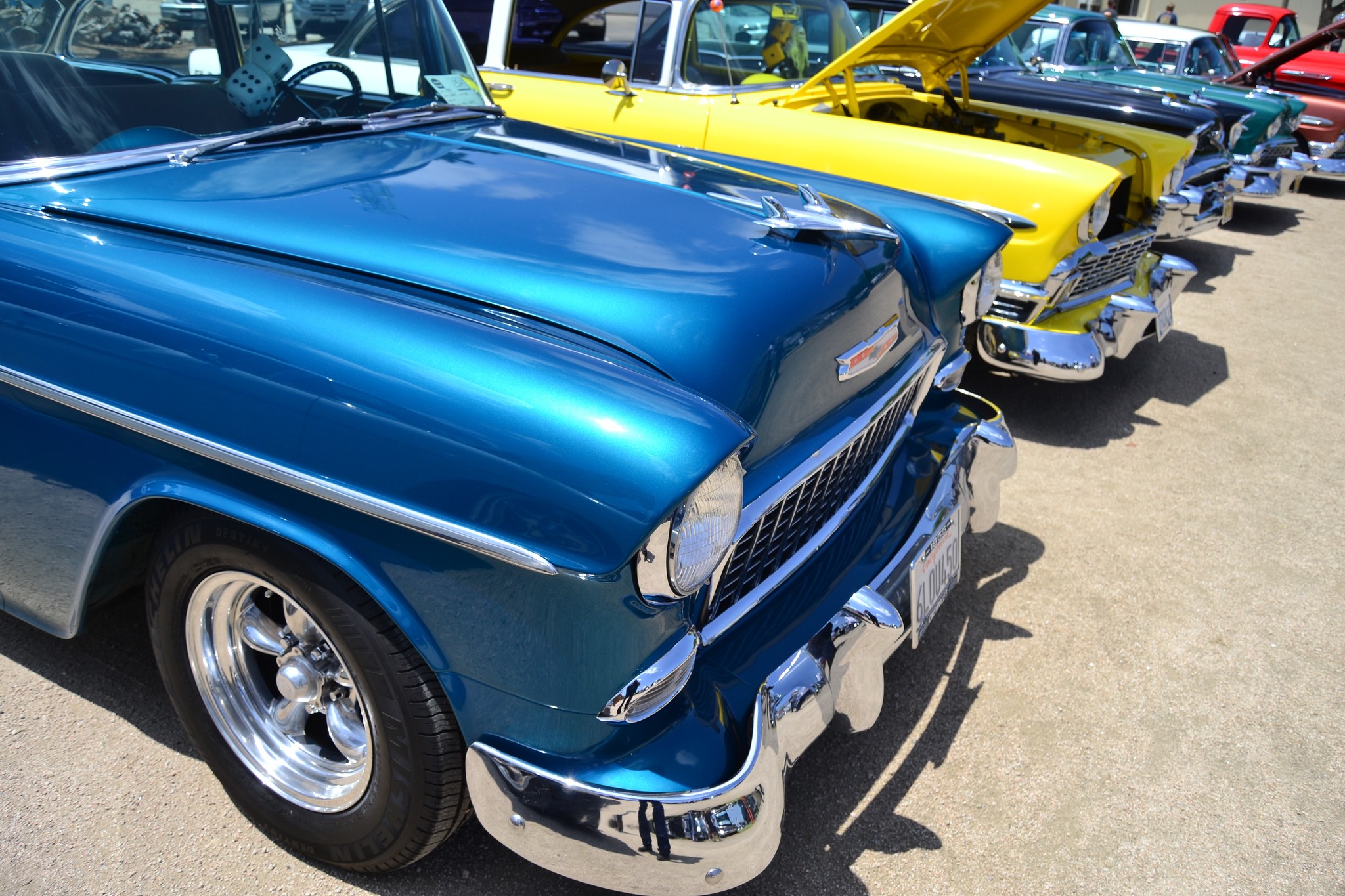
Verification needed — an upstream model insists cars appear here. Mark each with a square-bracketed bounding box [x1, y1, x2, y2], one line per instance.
[1, 0, 1018, 896]
[181, 0, 1345, 395]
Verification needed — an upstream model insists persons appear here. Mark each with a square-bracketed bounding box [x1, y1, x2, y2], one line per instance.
[1156, 4, 1178, 25]
[1098, 0, 1119, 18]
[735, 25, 752, 43]
